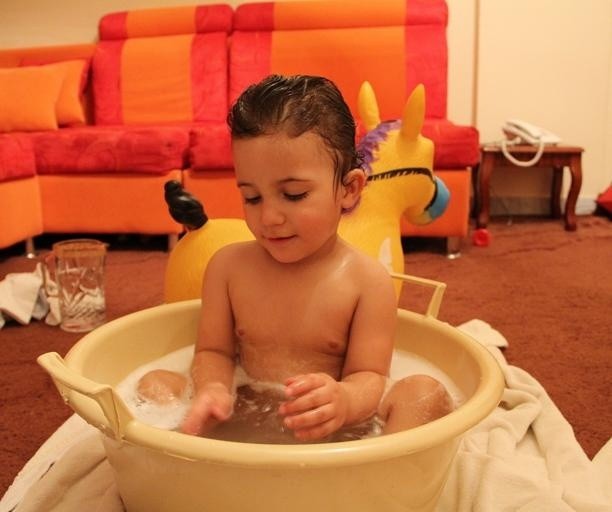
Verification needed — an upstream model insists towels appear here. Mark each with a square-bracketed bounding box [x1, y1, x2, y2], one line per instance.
[0, 263, 104, 327]
[14, 320, 612, 510]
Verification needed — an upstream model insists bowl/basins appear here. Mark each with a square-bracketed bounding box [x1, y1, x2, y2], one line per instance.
[35, 274, 509, 512]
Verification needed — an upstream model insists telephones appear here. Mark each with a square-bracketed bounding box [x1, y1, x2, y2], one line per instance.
[503, 120, 560, 147]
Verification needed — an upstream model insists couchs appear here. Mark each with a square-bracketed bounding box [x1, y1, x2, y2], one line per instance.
[0, 0, 481, 257]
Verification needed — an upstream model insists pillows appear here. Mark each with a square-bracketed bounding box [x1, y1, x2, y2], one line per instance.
[0, 58, 93, 132]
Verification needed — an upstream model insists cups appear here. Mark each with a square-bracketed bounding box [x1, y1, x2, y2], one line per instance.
[40, 236, 111, 336]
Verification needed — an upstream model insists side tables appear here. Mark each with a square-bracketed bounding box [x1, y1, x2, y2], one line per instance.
[471, 142, 585, 230]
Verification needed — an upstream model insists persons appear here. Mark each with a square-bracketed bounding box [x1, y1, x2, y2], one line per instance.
[133, 73, 456, 438]
